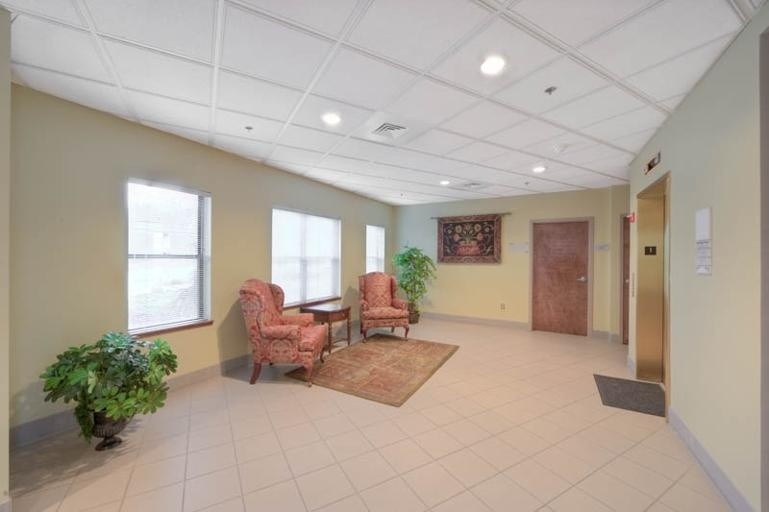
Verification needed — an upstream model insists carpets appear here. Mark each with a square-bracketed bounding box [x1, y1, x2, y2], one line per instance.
[284, 333, 460, 408]
[593, 374, 666, 418]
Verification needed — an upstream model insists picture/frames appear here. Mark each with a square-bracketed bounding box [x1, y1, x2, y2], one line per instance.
[436, 213, 502, 265]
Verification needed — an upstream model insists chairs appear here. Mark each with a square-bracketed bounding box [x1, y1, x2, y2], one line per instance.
[238, 278, 328, 388]
[358, 271, 410, 342]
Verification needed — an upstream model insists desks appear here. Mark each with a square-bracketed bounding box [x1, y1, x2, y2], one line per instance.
[300, 303, 352, 354]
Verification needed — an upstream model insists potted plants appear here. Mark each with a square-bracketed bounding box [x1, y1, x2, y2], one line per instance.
[39, 331, 178, 452]
[391, 245, 438, 324]
